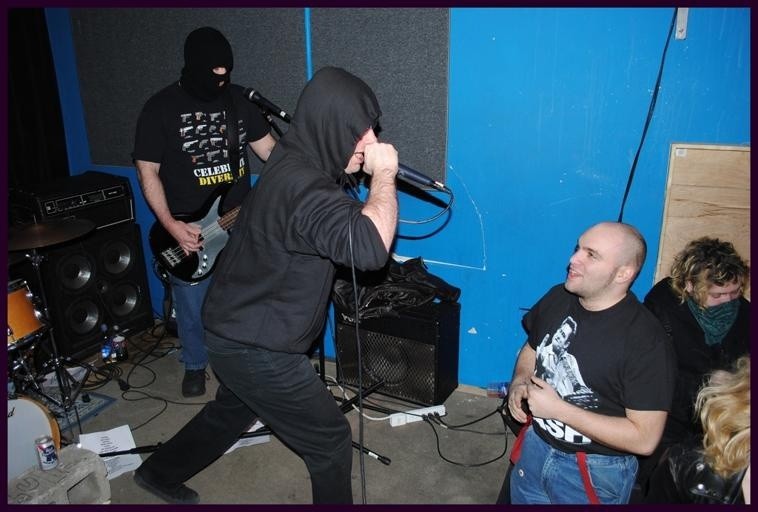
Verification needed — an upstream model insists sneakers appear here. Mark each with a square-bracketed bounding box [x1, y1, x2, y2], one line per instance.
[133, 469, 198, 503]
[181, 369, 209, 397]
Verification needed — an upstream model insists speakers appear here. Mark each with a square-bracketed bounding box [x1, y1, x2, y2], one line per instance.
[38, 224, 155, 364]
[334, 298, 460, 405]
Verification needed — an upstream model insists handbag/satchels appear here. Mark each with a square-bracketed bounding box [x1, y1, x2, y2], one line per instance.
[332, 258, 460, 323]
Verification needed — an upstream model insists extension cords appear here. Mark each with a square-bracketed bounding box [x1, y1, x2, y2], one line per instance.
[390, 407, 444, 427]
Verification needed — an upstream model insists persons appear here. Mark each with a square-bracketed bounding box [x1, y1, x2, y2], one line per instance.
[131, 26, 279, 398]
[132, 65, 401, 505]
[627, 234, 751, 504]
[640, 351, 750, 504]
[507, 221, 679, 505]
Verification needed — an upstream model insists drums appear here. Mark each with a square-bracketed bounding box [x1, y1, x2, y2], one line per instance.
[8, 394, 60, 481]
[7, 277, 48, 353]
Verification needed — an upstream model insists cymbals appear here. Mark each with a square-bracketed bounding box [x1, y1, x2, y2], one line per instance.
[7, 217, 96, 250]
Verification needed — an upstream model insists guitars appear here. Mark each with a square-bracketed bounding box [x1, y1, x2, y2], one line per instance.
[147, 177, 252, 287]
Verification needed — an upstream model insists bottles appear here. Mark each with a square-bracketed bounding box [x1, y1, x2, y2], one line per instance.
[99, 323, 129, 363]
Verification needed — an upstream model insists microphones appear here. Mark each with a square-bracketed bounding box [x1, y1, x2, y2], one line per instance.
[362, 153, 443, 195]
[244, 87, 295, 124]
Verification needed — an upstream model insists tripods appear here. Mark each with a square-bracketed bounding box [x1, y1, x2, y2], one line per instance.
[239, 327, 391, 468]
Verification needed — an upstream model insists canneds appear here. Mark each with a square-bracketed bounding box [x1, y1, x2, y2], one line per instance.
[35, 435, 58, 472]
